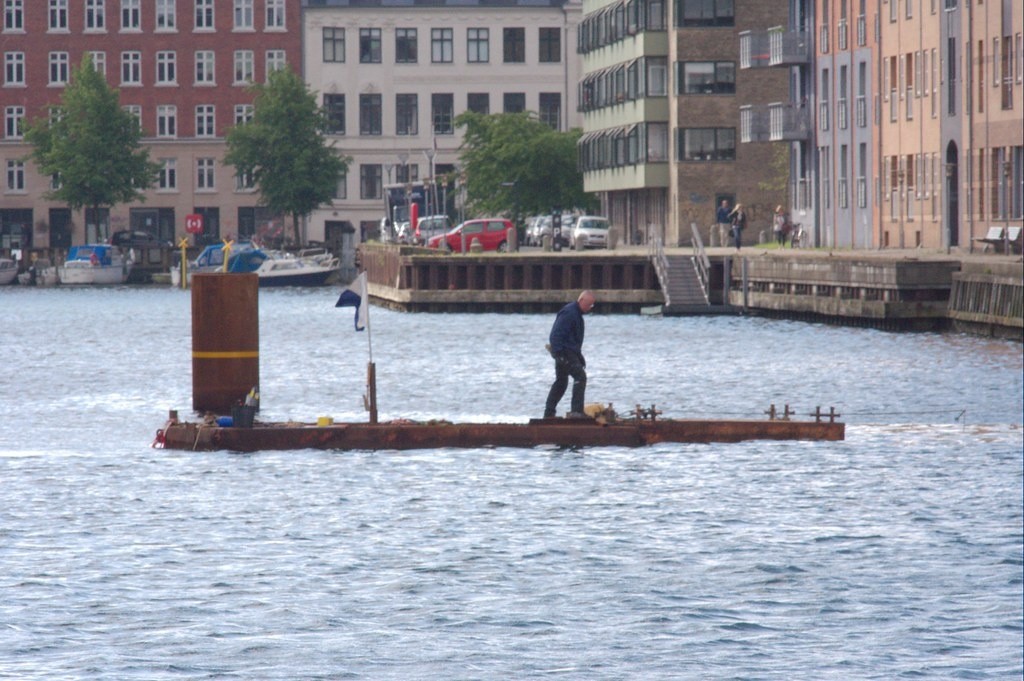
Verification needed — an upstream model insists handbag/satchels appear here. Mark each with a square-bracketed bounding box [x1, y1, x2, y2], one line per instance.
[779, 220, 792, 234]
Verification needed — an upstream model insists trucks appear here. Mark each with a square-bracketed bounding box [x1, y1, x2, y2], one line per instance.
[384, 183, 438, 243]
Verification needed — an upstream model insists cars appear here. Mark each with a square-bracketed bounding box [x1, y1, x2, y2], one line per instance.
[397, 214, 454, 246]
[428, 218, 516, 253]
[526, 214, 609, 249]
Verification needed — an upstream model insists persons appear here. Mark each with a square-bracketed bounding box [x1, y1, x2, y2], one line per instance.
[544, 290, 596, 418]
[716, 201, 731, 247]
[774, 205, 790, 250]
[728, 204, 748, 247]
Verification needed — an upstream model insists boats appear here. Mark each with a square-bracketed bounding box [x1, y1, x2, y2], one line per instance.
[153, 403, 847, 451]
[172, 244, 341, 290]
[58, 243, 134, 284]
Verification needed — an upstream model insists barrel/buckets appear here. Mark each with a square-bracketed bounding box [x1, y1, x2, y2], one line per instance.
[191, 273, 258, 409]
[233, 406, 255, 428]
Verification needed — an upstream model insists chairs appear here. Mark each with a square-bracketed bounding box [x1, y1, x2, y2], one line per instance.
[990, 227, 1022, 254]
[972, 227, 1002, 252]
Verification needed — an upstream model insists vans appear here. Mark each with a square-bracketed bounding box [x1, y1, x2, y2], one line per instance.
[380, 218, 392, 241]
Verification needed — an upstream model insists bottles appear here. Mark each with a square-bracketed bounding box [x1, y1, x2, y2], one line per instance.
[214, 416, 233, 427]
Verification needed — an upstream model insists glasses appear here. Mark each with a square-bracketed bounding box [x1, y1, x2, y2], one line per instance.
[584, 300, 594, 308]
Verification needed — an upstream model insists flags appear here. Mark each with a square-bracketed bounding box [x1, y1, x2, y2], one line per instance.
[335, 272, 368, 331]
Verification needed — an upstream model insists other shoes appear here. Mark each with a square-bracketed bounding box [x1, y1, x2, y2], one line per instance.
[566, 411, 593, 418]
